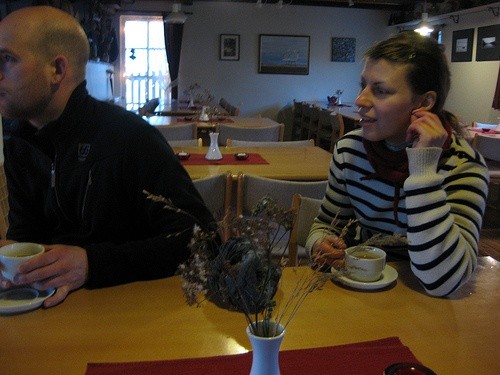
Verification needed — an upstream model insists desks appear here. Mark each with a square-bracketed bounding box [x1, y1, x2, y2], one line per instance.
[466, 127, 500, 161]
[174, 145, 332, 211]
[311, 100, 362, 130]
[144, 115, 279, 127]
[0, 255, 500, 375]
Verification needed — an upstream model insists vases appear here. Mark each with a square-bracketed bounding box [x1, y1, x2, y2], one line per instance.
[205, 133, 222, 160]
[199, 106, 211, 122]
[245, 321, 286, 375]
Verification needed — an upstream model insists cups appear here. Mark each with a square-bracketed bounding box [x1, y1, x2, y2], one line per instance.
[343, 246, 386, 282]
[0, 242, 45, 286]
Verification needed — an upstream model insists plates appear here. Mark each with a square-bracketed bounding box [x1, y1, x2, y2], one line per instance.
[0, 286, 55, 315]
[331, 264, 398, 290]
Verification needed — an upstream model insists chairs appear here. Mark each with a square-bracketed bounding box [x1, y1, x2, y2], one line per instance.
[161, 98, 344, 266]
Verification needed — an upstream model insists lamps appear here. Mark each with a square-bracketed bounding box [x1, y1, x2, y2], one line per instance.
[413, 0, 434, 32]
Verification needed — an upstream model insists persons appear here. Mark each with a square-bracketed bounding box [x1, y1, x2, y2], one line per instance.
[0, 6, 225, 308]
[305, 28, 490, 297]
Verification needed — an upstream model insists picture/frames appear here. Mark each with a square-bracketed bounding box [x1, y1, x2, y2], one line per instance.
[451, 27, 474, 62]
[476, 24, 500, 61]
[258, 34, 311, 75]
[331, 37, 355, 63]
[220, 34, 240, 61]
